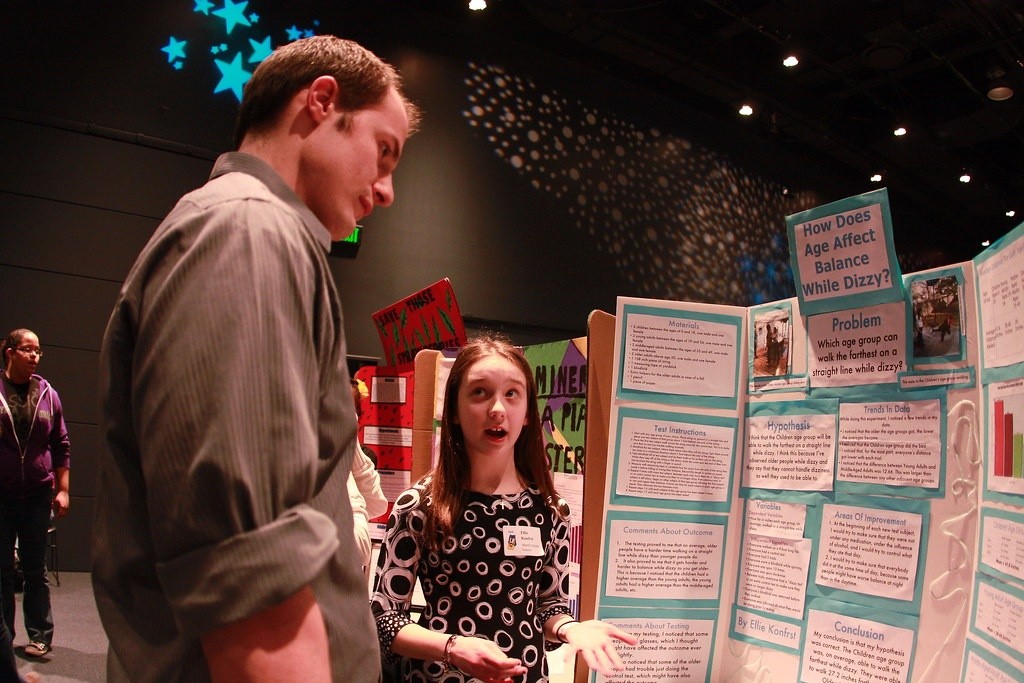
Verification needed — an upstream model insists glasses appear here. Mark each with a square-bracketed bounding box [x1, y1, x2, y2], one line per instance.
[8, 345, 42, 358]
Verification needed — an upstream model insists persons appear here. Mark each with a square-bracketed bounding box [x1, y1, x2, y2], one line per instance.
[92, 35, 420, 683]
[348, 437, 390, 564]
[0, 329, 71, 657]
[367, 338, 636, 683]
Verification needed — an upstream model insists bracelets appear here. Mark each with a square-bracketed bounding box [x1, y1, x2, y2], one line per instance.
[556, 620, 579, 644]
[442, 634, 456, 673]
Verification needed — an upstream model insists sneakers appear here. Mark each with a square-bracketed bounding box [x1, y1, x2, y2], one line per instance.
[24, 640, 49, 656]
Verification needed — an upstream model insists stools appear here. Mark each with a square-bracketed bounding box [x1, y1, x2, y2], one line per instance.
[47, 518, 61, 587]
